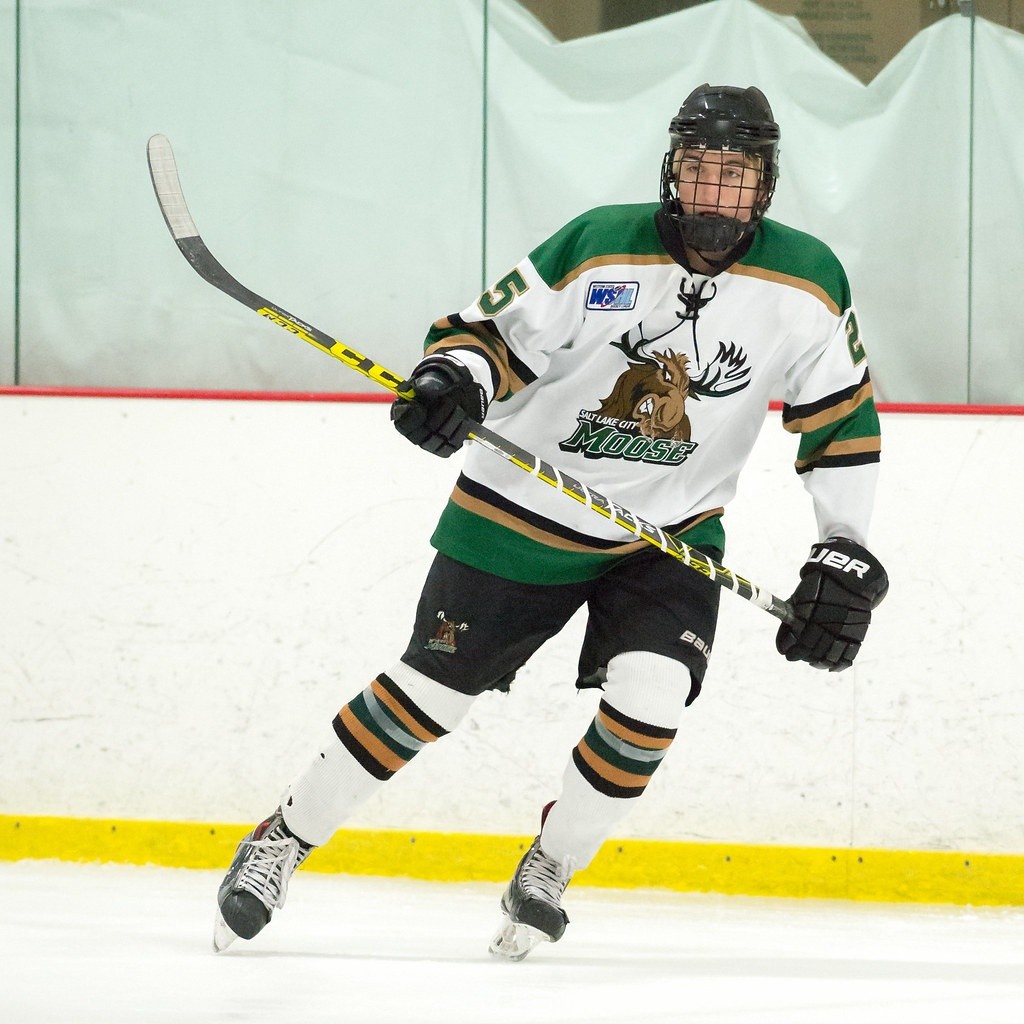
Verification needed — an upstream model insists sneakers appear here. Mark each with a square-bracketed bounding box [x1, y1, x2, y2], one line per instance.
[213, 805, 313, 953]
[488, 800, 577, 962]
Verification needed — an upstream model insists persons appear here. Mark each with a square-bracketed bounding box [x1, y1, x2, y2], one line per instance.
[210, 82, 889, 957]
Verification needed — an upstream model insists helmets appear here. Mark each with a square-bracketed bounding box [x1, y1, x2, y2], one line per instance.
[659, 83, 780, 252]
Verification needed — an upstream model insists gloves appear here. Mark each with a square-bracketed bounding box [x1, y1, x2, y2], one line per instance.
[776, 537, 889, 673]
[391, 352, 489, 459]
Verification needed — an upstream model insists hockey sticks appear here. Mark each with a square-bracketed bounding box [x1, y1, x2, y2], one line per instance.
[139, 131, 798, 631]
